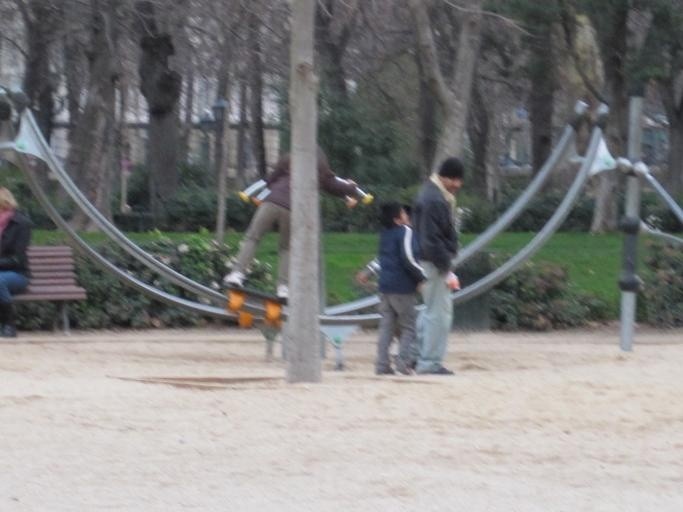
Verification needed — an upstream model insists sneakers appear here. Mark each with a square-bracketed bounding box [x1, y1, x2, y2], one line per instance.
[224, 272, 247, 286]
[277, 285, 288, 299]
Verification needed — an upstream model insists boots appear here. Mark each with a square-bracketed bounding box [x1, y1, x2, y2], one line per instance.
[1, 301, 17, 336]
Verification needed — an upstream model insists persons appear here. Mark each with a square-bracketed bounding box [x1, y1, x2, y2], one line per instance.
[356, 157, 467, 376]
[221, 137, 358, 299]
[0, 187, 32, 337]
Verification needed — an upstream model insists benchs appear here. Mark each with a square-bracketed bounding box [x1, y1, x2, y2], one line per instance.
[8, 243, 90, 337]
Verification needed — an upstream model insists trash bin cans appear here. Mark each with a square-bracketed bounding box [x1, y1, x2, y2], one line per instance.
[451, 251, 489, 334]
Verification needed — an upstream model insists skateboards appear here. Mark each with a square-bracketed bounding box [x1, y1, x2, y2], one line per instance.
[221, 281, 287, 328]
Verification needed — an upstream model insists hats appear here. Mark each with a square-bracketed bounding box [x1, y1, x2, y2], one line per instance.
[439, 157, 464, 178]
